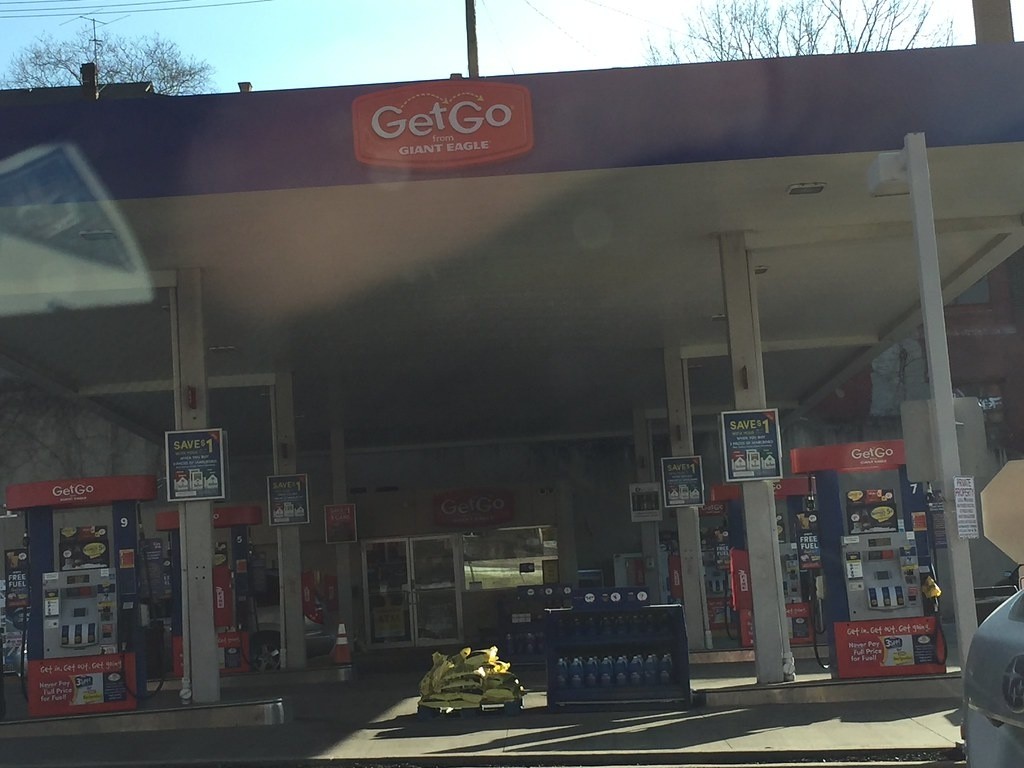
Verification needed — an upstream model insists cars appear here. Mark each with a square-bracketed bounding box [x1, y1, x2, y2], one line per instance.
[960, 587, 1024, 768]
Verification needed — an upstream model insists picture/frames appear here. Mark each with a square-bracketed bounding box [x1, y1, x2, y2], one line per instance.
[720, 408, 784, 484]
[266, 474, 311, 527]
[661, 455, 706, 508]
[324, 503, 358, 544]
[165, 428, 226, 502]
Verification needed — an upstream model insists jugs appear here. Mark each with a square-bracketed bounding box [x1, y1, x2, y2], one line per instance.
[556, 653, 673, 687]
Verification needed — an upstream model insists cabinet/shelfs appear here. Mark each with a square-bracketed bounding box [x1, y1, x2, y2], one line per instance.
[497, 583, 691, 713]
